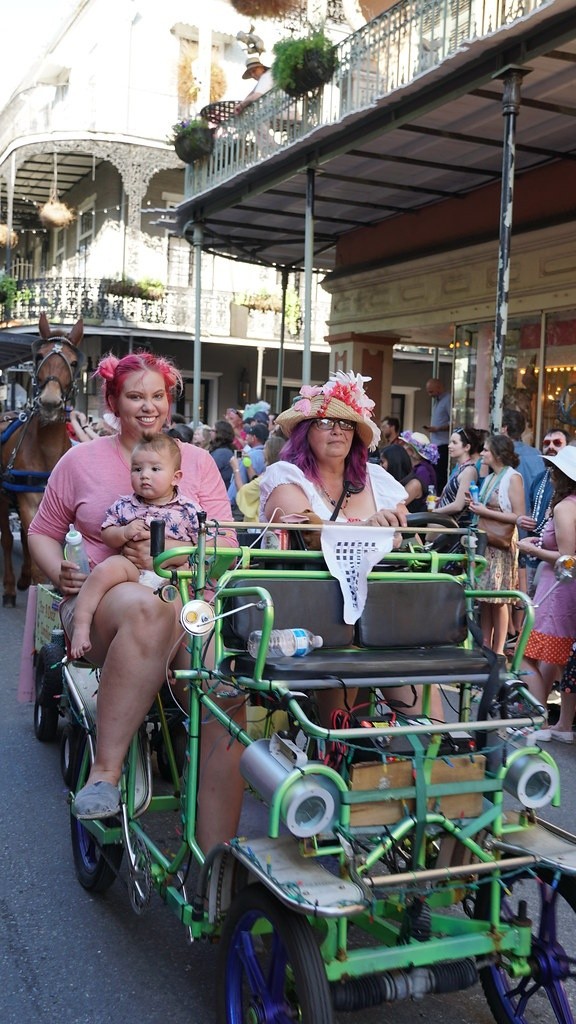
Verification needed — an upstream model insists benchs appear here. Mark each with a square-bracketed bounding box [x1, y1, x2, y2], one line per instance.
[214, 570, 534, 691]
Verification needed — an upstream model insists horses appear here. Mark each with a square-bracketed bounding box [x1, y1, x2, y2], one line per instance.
[0, 308, 84, 608]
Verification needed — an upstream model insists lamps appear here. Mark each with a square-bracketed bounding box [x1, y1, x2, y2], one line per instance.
[238, 367, 252, 408]
[83, 355, 97, 397]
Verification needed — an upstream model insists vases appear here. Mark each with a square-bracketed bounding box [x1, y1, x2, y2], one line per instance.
[174, 130, 214, 162]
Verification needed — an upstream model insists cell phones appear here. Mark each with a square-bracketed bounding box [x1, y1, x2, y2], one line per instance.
[423, 425, 429, 430]
[464, 491, 474, 505]
[235, 449, 243, 459]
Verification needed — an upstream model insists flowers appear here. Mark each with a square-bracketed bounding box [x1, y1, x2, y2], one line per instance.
[165, 116, 214, 146]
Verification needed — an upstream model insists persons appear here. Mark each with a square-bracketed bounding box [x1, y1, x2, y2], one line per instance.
[28, 354, 241, 919]
[71, 432, 216, 685]
[423, 379, 451, 496]
[256, 372, 444, 753]
[80, 403, 576, 746]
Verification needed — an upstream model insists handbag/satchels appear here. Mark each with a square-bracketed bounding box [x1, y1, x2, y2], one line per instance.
[425, 522, 447, 541]
[476, 504, 515, 550]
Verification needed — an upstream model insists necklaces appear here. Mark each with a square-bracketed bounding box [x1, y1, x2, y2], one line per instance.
[526, 522, 548, 561]
[455, 458, 474, 476]
[472, 466, 509, 524]
[318, 469, 351, 510]
[531, 466, 557, 533]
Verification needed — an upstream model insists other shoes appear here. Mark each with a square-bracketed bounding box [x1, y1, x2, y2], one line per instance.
[505, 724, 551, 741]
[504, 629, 521, 649]
[549, 726, 576, 744]
[74, 781, 120, 819]
[506, 702, 540, 718]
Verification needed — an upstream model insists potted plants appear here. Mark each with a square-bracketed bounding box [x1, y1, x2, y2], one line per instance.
[233, 287, 301, 335]
[0, 270, 16, 308]
[271, 21, 340, 97]
[97, 276, 166, 301]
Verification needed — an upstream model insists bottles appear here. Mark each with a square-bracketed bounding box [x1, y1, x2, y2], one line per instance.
[64, 523, 91, 577]
[426, 485, 437, 512]
[469, 480, 479, 505]
[248, 628, 324, 659]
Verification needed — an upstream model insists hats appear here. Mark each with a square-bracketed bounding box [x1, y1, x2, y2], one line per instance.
[104, 411, 122, 431]
[538, 445, 576, 481]
[243, 424, 269, 445]
[398, 430, 439, 465]
[274, 369, 381, 452]
[244, 411, 270, 426]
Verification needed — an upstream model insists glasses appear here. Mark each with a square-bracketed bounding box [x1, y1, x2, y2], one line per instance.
[542, 439, 567, 447]
[310, 417, 356, 430]
[453, 427, 468, 439]
